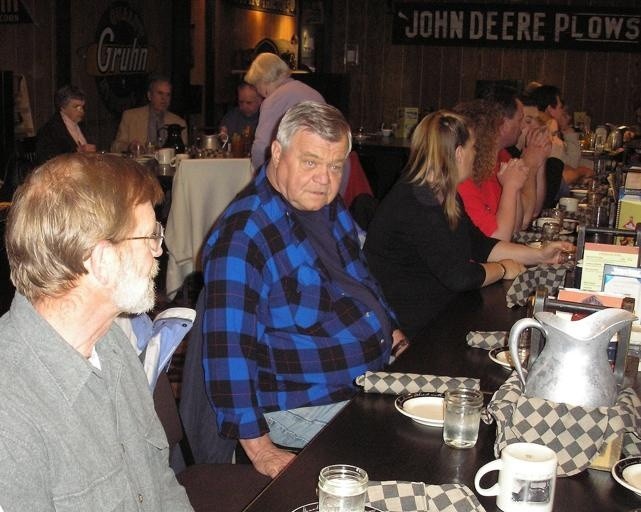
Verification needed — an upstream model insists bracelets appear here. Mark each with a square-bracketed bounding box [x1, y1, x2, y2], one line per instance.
[391, 338, 408, 356]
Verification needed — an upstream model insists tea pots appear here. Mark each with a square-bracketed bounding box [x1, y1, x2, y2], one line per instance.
[506, 307, 639, 410]
[155, 123, 187, 153]
[196, 133, 228, 152]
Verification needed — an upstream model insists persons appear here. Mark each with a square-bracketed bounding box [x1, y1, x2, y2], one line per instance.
[236, 50, 352, 203]
[108, 79, 190, 156]
[0, 150, 201, 512]
[358, 80, 600, 343]
[200, 99, 411, 479]
[215, 83, 260, 158]
[34, 82, 113, 170]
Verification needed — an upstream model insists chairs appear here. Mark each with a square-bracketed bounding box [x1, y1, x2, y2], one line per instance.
[121, 313, 280, 512]
[169, 158, 255, 307]
[348, 190, 377, 243]
[12, 134, 41, 187]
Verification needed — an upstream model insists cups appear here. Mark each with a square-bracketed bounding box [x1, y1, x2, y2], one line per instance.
[126, 140, 189, 170]
[474, 442, 557, 512]
[351, 127, 392, 142]
[441, 389, 483, 450]
[318, 464, 367, 511]
[529, 160, 621, 247]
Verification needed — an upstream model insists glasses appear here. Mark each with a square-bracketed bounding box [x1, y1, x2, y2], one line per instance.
[126, 221, 165, 252]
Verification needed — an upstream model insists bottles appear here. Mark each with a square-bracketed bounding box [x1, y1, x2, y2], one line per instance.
[579, 132, 613, 154]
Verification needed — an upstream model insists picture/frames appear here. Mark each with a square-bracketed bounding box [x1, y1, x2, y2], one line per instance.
[343, 42, 360, 66]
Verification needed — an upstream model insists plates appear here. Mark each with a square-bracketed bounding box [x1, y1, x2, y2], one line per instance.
[394, 391, 446, 426]
[489, 346, 517, 371]
[291, 501, 382, 512]
[610, 455, 641, 497]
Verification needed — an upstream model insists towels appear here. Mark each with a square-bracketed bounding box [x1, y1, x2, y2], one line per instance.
[531, 217, 580, 232]
[478, 368, 641, 477]
[363, 477, 485, 512]
[542, 207, 590, 222]
[512, 228, 574, 243]
[466, 329, 528, 351]
[355, 370, 481, 396]
[505, 263, 575, 307]
